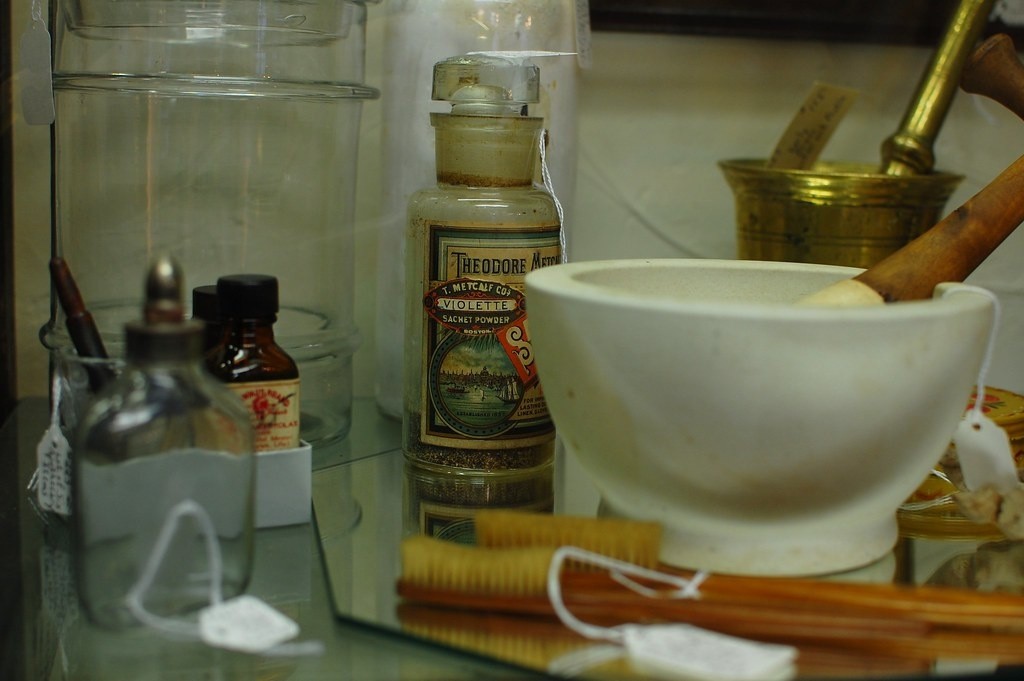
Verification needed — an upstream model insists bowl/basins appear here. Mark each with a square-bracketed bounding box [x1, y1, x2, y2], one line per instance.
[520, 254, 998, 579]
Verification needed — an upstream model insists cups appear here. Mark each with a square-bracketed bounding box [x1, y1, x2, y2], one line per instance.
[717, 157, 966, 269]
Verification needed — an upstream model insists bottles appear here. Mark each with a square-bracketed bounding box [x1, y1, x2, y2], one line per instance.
[63, 250, 301, 631]
[39, 0, 381, 454]
[404, 57, 564, 531]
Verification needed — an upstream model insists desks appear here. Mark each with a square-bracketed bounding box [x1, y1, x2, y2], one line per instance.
[0, 0, 1024, 681]
